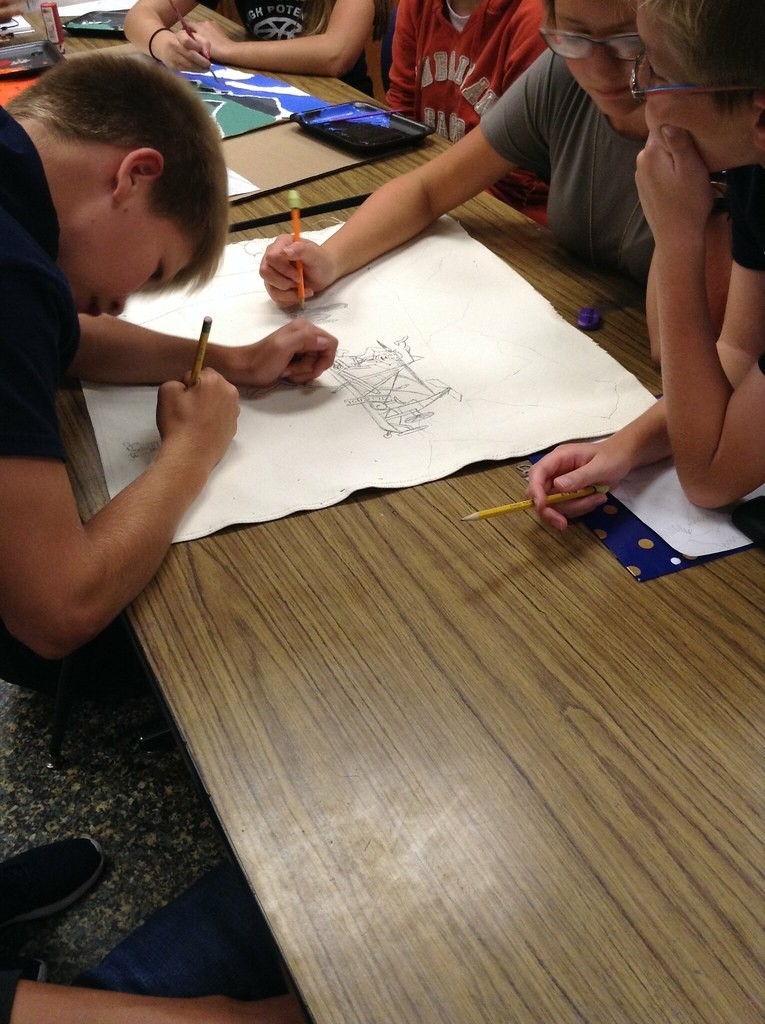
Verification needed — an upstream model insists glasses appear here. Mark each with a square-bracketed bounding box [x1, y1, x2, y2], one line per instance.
[536, 7, 644, 61]
[628, 47, 758, 104]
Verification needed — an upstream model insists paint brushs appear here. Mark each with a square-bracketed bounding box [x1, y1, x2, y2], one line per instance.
[311, 109, 404, 125]
[169, 0, 219, 84]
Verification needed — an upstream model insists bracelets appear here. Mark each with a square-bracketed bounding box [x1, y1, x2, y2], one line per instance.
[149, 28, 175, 61]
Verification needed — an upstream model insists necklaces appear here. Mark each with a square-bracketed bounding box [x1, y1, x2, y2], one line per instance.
[588, 108, 643, 279]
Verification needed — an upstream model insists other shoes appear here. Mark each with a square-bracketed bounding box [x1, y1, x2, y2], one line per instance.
[17, 959, 48, 983]
[0, 835, 106, 927]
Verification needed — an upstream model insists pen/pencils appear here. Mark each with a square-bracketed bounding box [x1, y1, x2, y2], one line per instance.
[461, 486, 609, 523]
[288, 190, 306, 310]
[186, 317, 212, 388]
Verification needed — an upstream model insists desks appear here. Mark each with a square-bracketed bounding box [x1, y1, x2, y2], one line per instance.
[0, 2, 764, 1024]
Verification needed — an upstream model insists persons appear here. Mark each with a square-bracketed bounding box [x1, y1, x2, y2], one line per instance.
[385, 0, 551, 225]
[0, 54, 338, 976]
[528, 0, 765, 532]
[260, 0, 732, 363]
[124, 0, 378, 101]
